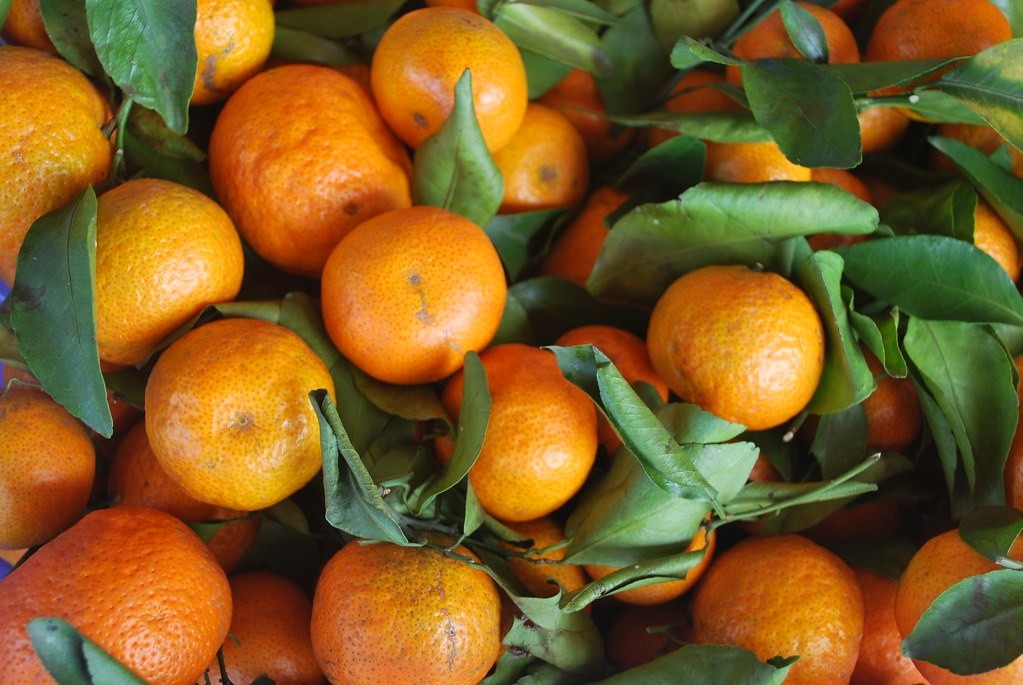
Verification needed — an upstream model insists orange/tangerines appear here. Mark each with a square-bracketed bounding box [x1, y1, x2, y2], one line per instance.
[2, 1, 1022, 684]
